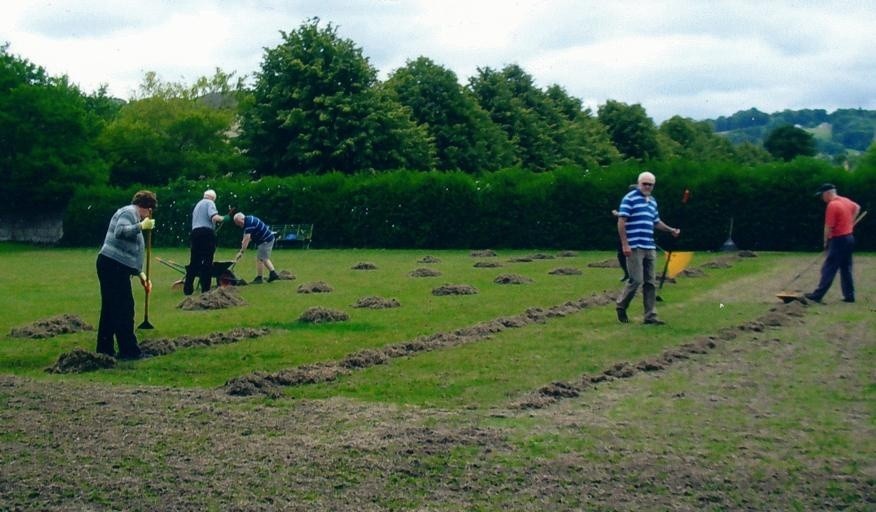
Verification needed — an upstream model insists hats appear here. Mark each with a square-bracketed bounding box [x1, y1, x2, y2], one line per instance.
[815, 183, 836, 197]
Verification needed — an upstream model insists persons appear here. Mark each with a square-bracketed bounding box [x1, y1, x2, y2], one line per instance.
[233, 212, 279, 283]
[805, 183, 862, 303]
[612, 209, 630, 282]
[615, 172, 681, 325]
[183, 190, 232, 295]
[95, 189, 157, 361]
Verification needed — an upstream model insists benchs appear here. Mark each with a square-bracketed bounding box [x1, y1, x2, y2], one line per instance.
[254, 223, 314, 249]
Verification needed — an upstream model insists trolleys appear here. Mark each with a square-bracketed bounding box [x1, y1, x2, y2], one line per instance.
[155, 257, 237, 289]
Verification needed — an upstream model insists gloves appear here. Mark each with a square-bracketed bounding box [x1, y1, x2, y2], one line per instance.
[141, 216, 155, 230]
[223, 215, 230, 223]
[139, 272, 152, 294]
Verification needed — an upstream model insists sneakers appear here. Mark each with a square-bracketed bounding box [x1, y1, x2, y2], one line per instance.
[804, 291, 855, 305]
[615, 306, 665, 325]
[249, 274, 281, 284]
